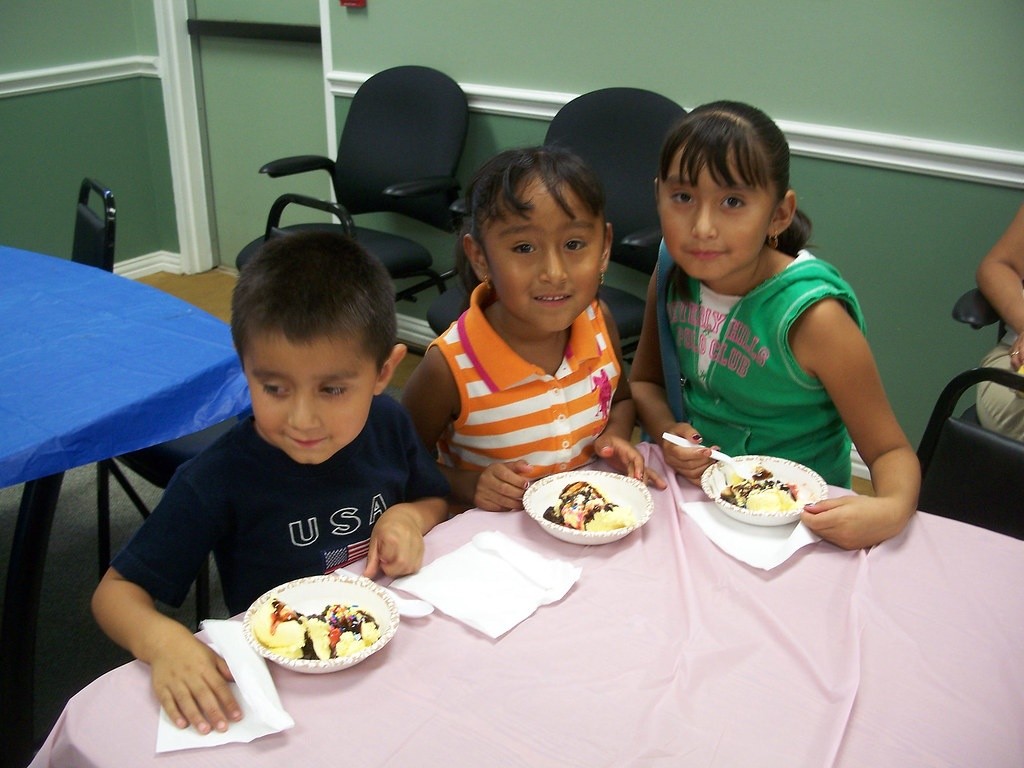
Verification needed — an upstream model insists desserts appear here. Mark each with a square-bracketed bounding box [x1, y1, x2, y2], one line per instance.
[542, 481, 632, 529]
[252, 593, 379, 661]
[718, 463, 800, 512]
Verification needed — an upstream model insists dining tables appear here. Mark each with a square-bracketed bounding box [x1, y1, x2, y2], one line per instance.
[3, 240, 255, 768]
[26, 473, 1024, 768]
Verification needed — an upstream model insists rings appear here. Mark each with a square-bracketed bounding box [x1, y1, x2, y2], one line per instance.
[1010, 351, 1021, 358]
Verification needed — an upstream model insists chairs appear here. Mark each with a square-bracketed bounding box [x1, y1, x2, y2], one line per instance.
[425, 87, 690, 365]
[236, 63, 472, 299]
[914, 286, 1024, 424]
[95, 190, 353, 652]
[7, 171, 122, 675]
[914, 365, 1023, 542]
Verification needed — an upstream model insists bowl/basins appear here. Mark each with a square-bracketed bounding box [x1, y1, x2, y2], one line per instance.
[242, 575, 402, 675]
[522, 469, 654, 547]
[700, 454, 828, 528]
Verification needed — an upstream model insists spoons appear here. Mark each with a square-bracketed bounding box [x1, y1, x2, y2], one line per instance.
[334, 566, 435, 619]
[662, 431, 754, 481]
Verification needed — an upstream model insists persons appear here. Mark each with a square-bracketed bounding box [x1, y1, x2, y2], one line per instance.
[399, 145, 668, 512]
[92, 227, 453, 733]
[630, 99, 922, 548]
[977, 200, 1024, 445]
